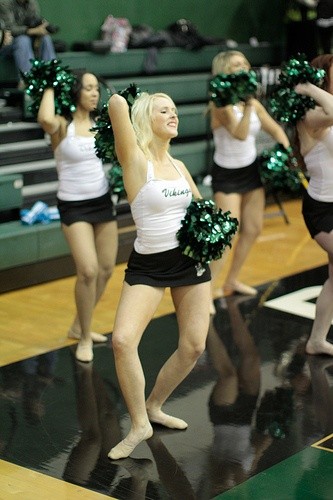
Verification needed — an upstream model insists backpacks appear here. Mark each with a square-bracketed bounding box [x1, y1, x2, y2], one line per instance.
[95, 15, 206, 51]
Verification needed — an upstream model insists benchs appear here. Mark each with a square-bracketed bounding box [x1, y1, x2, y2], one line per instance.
[0, 39, 305, 275]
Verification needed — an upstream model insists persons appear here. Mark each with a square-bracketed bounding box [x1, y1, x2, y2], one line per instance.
[223, 0, 333, 67]
[106, 91, 211, 459]
[206, 49, 292, 314]
[36, 68, 118, 362]
[0, 0, 54, 83]
[0, 292, 333, 500]
[295, 54, 333, 356]
